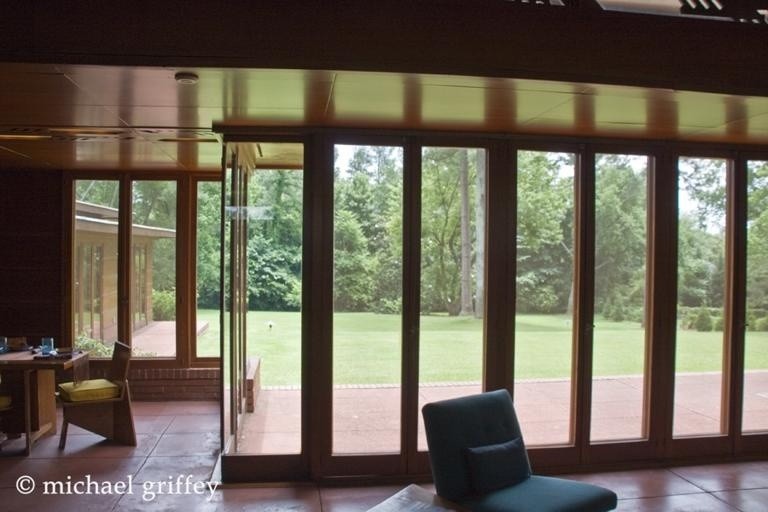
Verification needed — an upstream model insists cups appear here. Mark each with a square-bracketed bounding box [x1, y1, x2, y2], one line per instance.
[42, 338, 54, 354]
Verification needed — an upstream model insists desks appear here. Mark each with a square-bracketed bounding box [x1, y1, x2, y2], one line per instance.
[0, 350, 90, 457]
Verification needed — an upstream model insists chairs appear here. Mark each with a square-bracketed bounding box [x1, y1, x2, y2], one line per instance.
[54, 342, 137, 452]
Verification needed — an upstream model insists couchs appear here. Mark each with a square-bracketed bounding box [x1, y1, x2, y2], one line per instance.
[422, 389, 617, 512]
